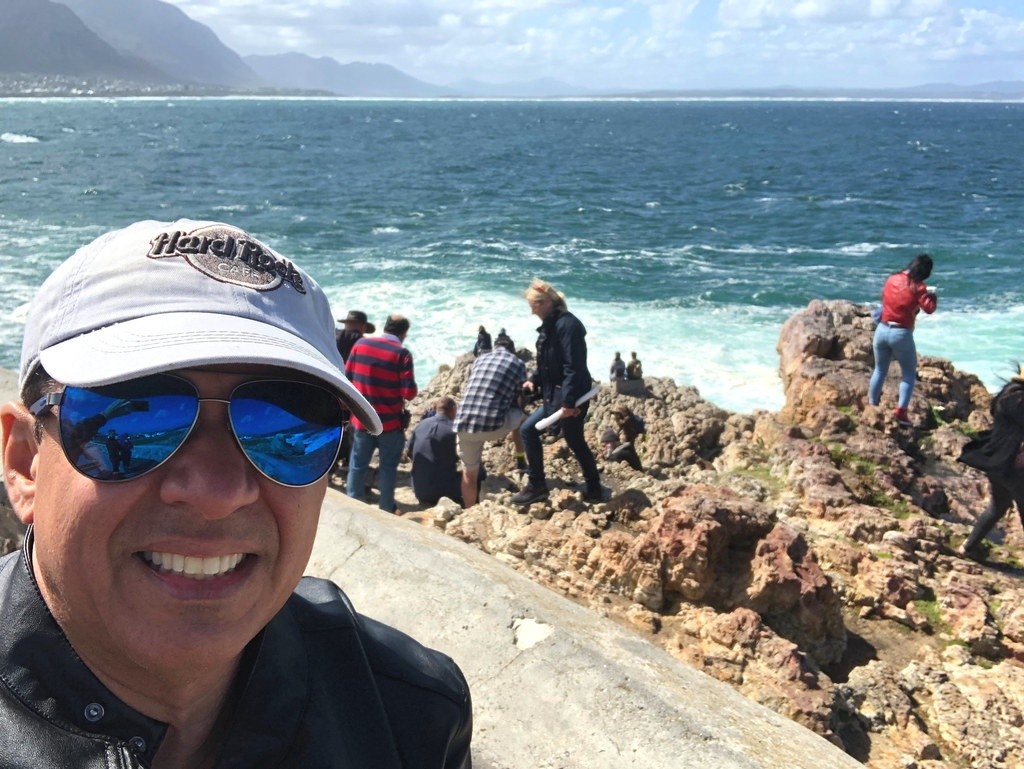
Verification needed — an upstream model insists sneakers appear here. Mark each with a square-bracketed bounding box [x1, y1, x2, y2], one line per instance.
[575, 482, 602, 501]
[958, 537, 987, 558]
[515, 451, 528, 470]
[510, 482, 550, 505]
[891, 406, 910, 426]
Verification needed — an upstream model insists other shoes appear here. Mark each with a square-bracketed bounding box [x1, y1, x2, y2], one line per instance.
[393, 505, 401, 516]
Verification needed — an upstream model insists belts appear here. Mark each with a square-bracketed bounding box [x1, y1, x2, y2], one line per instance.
[880, 321, 908, 328]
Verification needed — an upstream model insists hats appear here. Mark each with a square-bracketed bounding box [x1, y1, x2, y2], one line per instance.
[599, 428, 620, 441]
[19, 218, 382, 437]
[337, 310, 375, 334]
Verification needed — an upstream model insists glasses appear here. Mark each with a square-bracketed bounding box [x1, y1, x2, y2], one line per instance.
[31, 373, 350, 488]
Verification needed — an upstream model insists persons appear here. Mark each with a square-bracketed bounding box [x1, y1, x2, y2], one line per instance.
[608, 352, 643, 380]
[405, 397, 488, 508]
[474, 325, 509, 357]
[1, 217, 473, 769]
[510, 279, 602, 505]
[866, 252, 938, 425]
[337, 315, 417, 515]
[326, 310, 377, 483]
[451, 337, 530, 509]
[953, 362, 1023, 558]
[602, 403, 644, 471]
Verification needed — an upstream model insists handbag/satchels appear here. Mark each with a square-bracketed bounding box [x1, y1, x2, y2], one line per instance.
[1009, 439, 1023, 469]
[871, 305, 884, 324]
